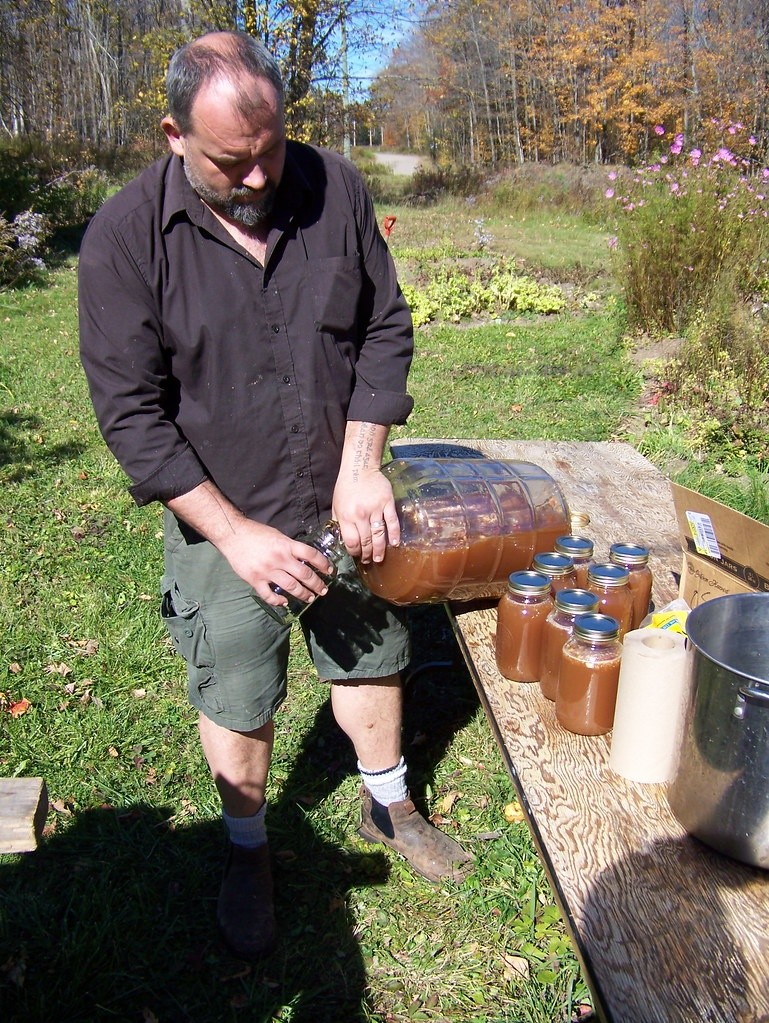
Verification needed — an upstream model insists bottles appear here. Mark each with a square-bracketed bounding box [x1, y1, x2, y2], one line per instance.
[496, 535, 653, 736]
[252, 525, 346, 624]
[328, 457, 573, 606]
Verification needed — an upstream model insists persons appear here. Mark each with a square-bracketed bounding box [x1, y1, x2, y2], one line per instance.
[77, 30, 478, 957]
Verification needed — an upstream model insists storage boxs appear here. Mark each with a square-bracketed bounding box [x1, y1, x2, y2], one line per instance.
[669, 481, 769, 611]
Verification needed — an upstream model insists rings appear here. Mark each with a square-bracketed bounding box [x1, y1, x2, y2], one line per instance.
[372, 520, 385, 527]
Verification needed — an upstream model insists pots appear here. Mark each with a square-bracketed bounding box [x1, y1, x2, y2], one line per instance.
[669, 592, 769, 872]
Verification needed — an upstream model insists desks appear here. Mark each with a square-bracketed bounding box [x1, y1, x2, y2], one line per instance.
[391, 438, 769, 1023]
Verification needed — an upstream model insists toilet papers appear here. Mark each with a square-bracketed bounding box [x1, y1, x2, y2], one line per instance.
[609, 627, 690, 783]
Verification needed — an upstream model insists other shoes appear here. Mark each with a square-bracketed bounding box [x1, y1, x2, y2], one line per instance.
[216, 839, 275, 964]
[355, 784, 478, 884]
